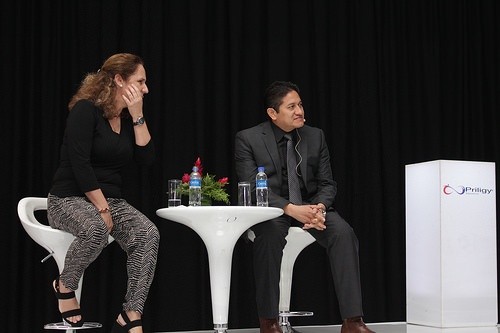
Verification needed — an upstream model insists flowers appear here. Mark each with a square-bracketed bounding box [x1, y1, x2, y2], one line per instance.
[167, 157, 231, 206]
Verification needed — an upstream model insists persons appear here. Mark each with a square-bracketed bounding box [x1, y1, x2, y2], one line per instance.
[46, 53, 160, 333]
[235, 81, 376, 333]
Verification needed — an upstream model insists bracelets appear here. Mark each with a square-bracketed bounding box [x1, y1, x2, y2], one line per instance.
[98, 204, 113, 213]
[321, 209, 326, 217]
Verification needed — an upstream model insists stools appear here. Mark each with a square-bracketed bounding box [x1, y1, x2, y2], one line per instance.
[246, 227, 317, 333]
[17, 197, 114, 333]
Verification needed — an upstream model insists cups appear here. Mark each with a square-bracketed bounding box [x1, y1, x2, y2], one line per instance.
[167, 179, 182, 208]
[237, 181, 251, 206]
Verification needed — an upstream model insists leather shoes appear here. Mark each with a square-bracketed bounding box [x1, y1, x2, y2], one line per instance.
[260, 316, 299, 333]
[340, 316, 376, 333]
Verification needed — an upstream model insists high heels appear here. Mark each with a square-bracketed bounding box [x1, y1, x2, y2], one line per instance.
[115, 312, 144, 333]
[52, 278, 84, 327]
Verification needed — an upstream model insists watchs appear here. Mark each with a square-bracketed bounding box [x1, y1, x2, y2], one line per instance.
[133, 117, 146, 126]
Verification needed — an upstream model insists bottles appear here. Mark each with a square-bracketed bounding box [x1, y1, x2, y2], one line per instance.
[256, 167, 268, 207]
[188, 167, 201, 206]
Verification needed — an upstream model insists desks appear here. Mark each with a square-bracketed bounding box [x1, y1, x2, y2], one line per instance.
[156, 205, 284, 333]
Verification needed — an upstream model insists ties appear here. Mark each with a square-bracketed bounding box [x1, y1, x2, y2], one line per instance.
[283, 134, 302, 205]
